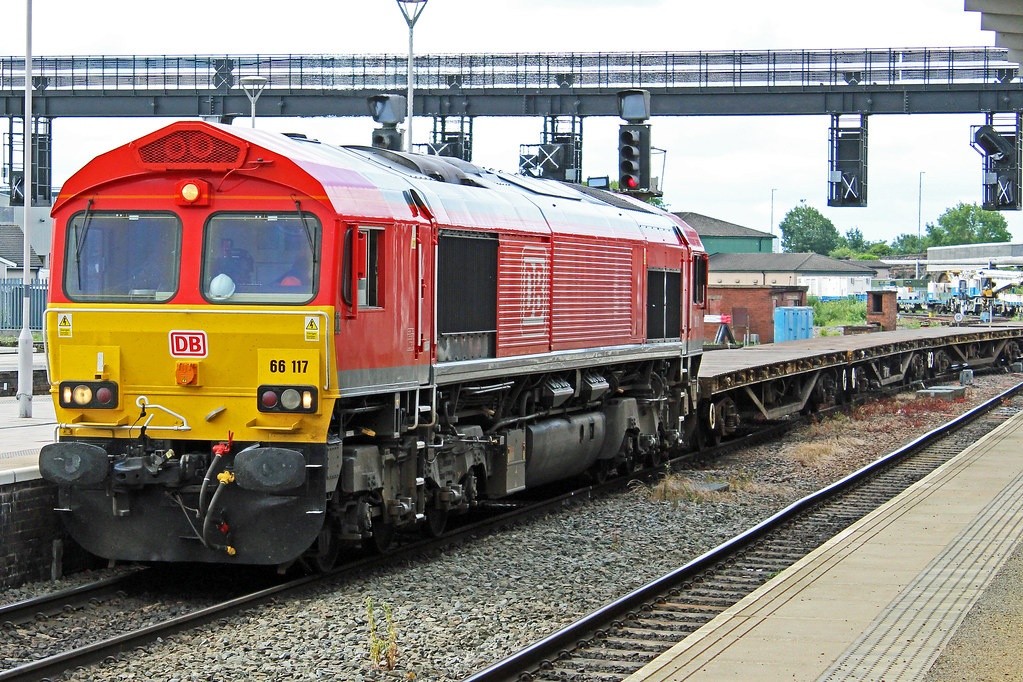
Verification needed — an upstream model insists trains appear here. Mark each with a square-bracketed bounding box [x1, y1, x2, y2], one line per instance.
[36, 118, 1023, 584]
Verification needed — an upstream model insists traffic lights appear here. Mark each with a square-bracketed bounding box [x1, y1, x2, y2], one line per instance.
[619, 127, 650, 190]
[372, 126, 401, 150]
